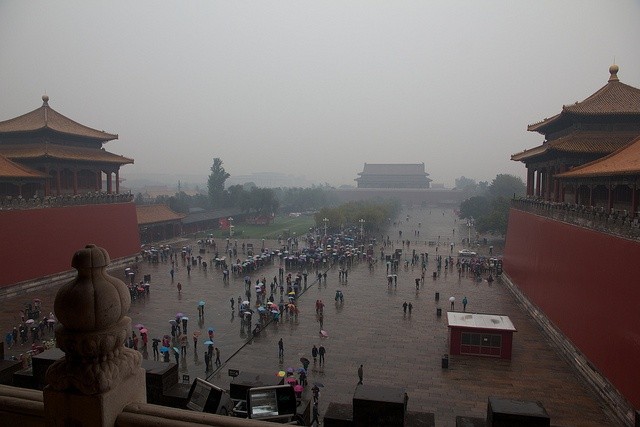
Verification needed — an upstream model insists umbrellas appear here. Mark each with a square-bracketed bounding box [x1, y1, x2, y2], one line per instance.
[242, 300, 295, 315]
[275, 371, 287, 378]
[320, 329, 329, 338]
[180, 317, 189, 320]
[34, 299, 40, 303]
[203, 341, 214, 344]
[284, 378, 297, 382]
[311, 382, 324, 387]
[300, 358, 309, 364]
[135, 324, 144, 327]
[167, 320, 177, 325]
[173, 347, 180, 355]
[207, 328, 215, 331]
[298, 368, 307, 374]
[139, 328, 148, 334]
[175, 313, 184, 316]
[152, 337, 161, 342]
[286, 368, 296, 372]
[336, 289, 341, 292]
[449, 297, 455, 301]
[160, 347, 170, 352]
[162, 335, 171, 339]
[294, 384, 304, 392]
[201, 306, 204, 316]
[198, 301, 205, 305]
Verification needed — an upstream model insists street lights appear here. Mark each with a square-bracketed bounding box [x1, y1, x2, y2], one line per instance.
[467, 221, 473, 243]
[359, 218, 365, 238]
[228, 216, 234, 235]
[323, 217, 329, 234]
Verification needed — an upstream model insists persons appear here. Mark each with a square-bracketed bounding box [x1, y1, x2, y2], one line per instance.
[165, 337, 169, 346]
[181, 246, 207, 274]
[32, 312, 58, 355]
[159, 351, 165, 362]
[296, 392, 301, 398]
[463, 296, 467, 311]
[170, 270, 174, 280]
[141, 243, 177, 269]
[421, 272, 424, 283]
[208, 344, 213, 359]
[311, 402, 321, 427]
[32, 297, 41, 312]
[174, 353, 179, 365]
[336, 292, 339, 301]
[357, 364, 363, 385]
[5, 304, 32, 350]
[177, 282, 182, 294]
[451, 301, 455, 310]
[211, 248, 280, 267]
[164, 351, 169, 362]
[230, 298, 235, 310]
[152, 340, 158, 357]
[208, 331, 213, 342]
[312, 345, 318, 363]
[408, 303, 413, 314]
[303, 359, 308, 371]
[133, 334, 138, 350]
[175, 318, 181, 332]
[197, 305, 202, 317]
[193, 333, 198, 351]
[125, 268, 150, 302]
[129, 337, 133, 348]
[215, 348, 221, 364]
[178, 333, 187, 355]
[304, 227, 360, 245]
[143, 334, 148, 346]
[245, 267, 327, 296]
[339, 267, 348, 284]
[300, 373, 304, 385]
[416, 277, 420, 290]
[172, 325, 175, 336]
[319, 300, 324, 315]
[339, 293, 343, 303]
[280, 244, 367, 267]
[205, 352, 210, 371]
[316, 300, 319, 314]
[183, 320, 187, 333]
[223, 267, 245, 281]
[403, 302, 407, 313]
[404, 392, 409, 409]
[162, 337, 165, 346]
[237, 295, 299, 336]
[319, 345, 325, 363]
[406, 249, 502, 273]
[141, 328, 144, 339]
[311, 385, 319, 404]
[8, 351, 31, 368]
[278, 339, 284, 356]
[366, 243, 402, 289]
